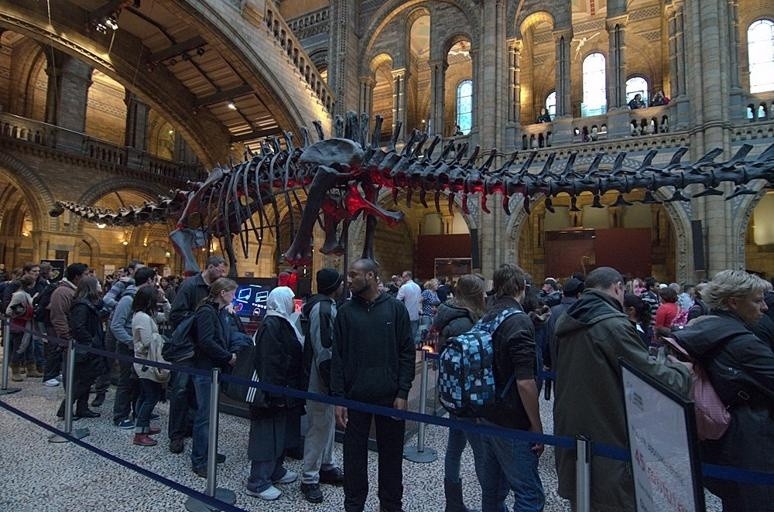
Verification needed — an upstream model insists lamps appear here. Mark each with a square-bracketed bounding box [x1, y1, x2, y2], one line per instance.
[88, 10, 122, 35]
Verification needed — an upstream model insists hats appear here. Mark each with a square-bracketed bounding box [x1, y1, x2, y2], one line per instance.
[317, 269, 343, 295]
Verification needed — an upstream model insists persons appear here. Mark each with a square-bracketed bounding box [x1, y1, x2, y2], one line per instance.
[1, 257, 354, 503]
[411, 90, 669, 139]
[378, 262, 774, 512]
[330, 258, 417, 511]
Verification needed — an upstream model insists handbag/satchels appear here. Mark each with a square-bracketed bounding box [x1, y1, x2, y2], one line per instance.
[225, 344, 270, 407]
[62, 337, 96, 363]
[98, 279, 127, 309]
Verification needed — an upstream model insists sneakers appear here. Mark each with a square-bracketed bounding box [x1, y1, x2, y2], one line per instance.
[216, 454, 226, 463]
[192, 468, 207, 478]
[272, 470, 297, 484]
[320, 467, 344, 485]
[92, 394, 105, 407]
[169, 439, 184, 453]
[150, 414, 161, 420]
[300, 480, 323, 503]
[246, 485, 282, 501]
[133, 434, 157, 446]
[117, 419, 134, 429]
[149, 428, 161, 434]
[42, 379, 59, 387]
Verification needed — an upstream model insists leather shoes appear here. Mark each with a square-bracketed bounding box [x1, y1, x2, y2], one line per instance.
[76, 408, 100, 418]
[57, 403, 65, 416]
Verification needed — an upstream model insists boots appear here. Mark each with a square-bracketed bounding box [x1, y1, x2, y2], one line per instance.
[444, 478, 480, 512]
[26, 360, 42, 377]
[9, 362, 24, 381]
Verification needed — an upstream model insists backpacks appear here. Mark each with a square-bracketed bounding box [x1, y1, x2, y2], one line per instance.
[564, 278, 584, 297]
[438, 307, 525, 418]
[32, 284, 58, 322]
[161, 308, 219, 365]
[663, 336, 750, 441]
